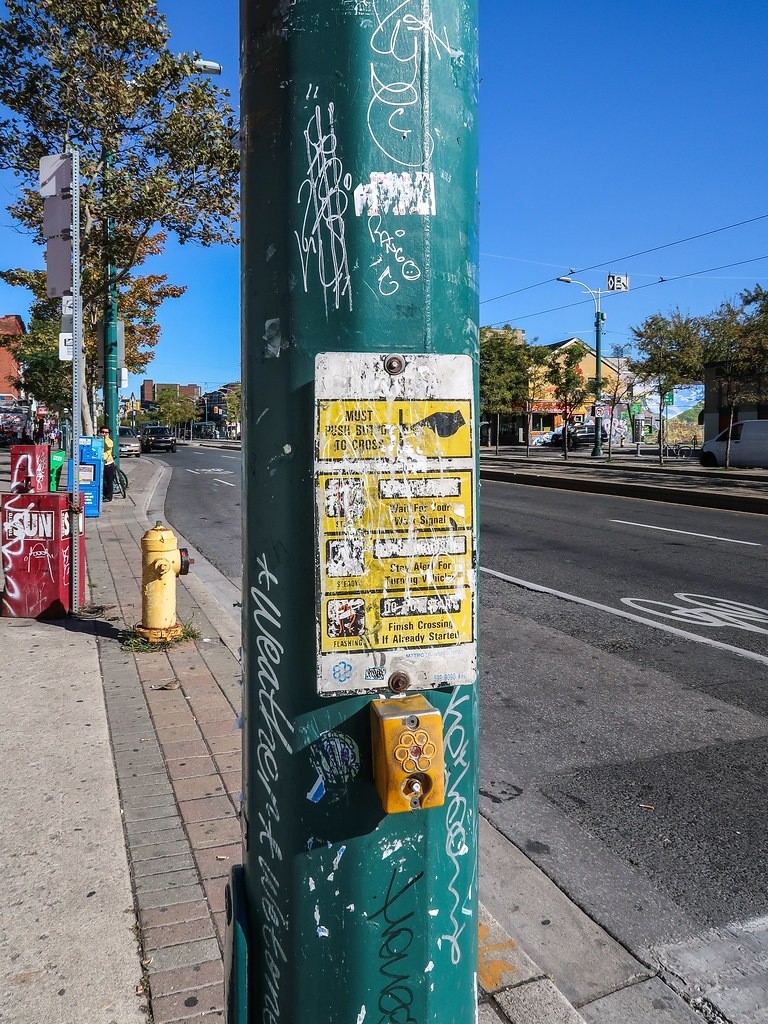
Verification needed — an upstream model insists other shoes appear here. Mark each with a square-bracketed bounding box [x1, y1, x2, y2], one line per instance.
[103, 499, 111, 502]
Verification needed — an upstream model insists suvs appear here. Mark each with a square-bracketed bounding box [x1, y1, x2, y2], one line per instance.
[140, 426, 177, 454]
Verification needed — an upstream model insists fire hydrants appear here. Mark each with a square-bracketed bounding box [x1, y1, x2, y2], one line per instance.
[136, 520, 196, 640]
[621, 435, 625, 448]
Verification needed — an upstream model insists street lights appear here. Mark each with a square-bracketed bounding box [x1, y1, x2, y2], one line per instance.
[199, 397, 208, 427]
[556, 276, 605, 456]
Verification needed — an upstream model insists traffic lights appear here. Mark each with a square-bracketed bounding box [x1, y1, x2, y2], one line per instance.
[126, 403, 130, 410]
[214, 406, 218, 413]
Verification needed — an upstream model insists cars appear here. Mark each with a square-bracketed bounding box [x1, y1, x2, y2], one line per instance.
[551, 424, 608, 451]
[238, 432, 241, 440]
[102, 56, 224, 465]
[116, 425, 140, 458]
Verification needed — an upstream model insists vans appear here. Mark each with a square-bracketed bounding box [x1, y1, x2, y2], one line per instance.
[699, 420, 768, 469]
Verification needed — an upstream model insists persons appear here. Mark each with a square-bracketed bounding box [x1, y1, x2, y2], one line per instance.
[48, 430, 56, 448]
[34, 429, 41, 446]
[100, 426, 115, 502]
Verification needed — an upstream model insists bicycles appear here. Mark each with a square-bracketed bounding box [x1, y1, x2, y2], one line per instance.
[113, 468, 129, 499]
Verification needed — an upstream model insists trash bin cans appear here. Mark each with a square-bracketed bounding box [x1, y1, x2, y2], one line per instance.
[50, 451, 65, 491]
[0, 492, 85, 618]
[67, 436, 103, 517]
[10, 445, 51, 493]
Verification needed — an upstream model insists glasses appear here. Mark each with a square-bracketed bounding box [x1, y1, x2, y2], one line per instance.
[101, 431, 110, 433]
